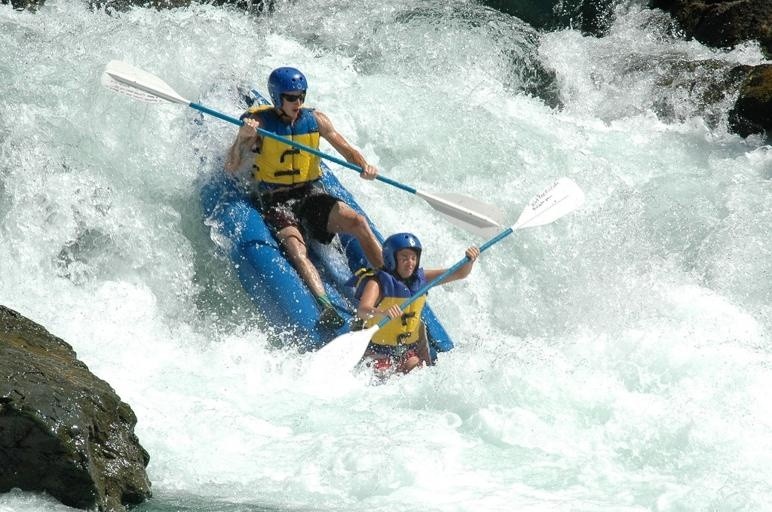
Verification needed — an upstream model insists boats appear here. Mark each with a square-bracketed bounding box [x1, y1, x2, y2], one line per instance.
[190, 86, 455, 356]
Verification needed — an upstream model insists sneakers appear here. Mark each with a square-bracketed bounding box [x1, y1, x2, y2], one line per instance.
[319, 307, 346, 330]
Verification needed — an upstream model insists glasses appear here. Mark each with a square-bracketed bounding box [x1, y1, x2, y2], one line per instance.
[281, 92, 306, 102]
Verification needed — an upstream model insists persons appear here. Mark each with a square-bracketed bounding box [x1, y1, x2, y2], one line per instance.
[226, 67, 386, 330]
[358, 233, 482, 387]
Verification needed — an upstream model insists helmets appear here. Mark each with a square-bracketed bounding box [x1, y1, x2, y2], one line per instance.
[267, 66, 307, 108]
[382, 233, 422, 277]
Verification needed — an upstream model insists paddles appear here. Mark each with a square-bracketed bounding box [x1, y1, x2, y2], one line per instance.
[316, 176, 586, 369]
[99, 59, 504, 241]
